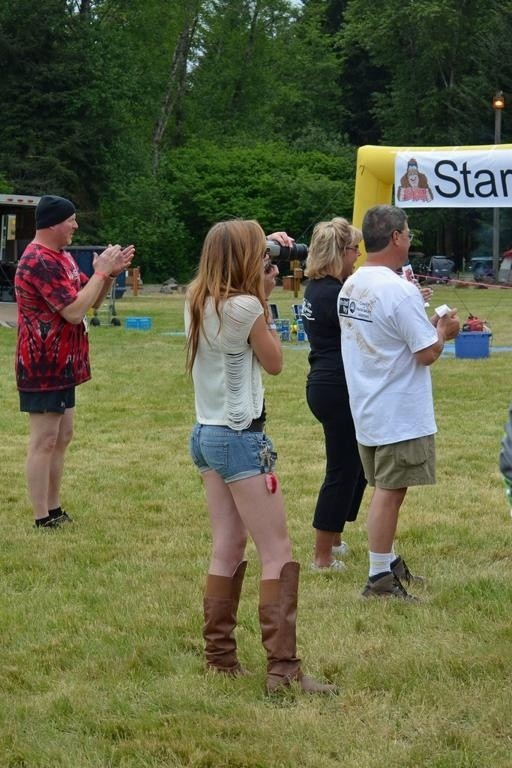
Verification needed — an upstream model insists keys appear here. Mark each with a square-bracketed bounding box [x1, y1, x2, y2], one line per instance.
[257, 440, 274, 468]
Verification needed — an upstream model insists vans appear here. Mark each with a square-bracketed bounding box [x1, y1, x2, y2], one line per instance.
[63, 243, 126, 300]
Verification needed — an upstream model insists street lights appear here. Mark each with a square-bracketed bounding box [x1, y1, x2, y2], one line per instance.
[490, 91, 506, 281]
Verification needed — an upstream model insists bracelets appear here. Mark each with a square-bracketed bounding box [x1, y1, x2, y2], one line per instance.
[267, 322, 278, 330]
[94, 270, 108, 280]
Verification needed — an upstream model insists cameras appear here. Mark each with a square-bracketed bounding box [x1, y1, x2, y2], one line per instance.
[265, 236, 309, 263]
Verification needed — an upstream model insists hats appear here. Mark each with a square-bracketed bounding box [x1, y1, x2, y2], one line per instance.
[35, 195, 76, 229]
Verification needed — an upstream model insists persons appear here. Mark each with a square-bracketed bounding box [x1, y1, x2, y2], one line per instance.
[303, 218, 362, 570]
[14, 196, 137, 528]
[183, 218, 341, 694]
[337, 204, 458, 603]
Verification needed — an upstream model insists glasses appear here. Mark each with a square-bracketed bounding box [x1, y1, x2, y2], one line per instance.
[396, 229, 414, 241]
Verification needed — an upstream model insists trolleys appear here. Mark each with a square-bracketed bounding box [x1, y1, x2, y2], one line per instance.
[88, 275, 120, 330]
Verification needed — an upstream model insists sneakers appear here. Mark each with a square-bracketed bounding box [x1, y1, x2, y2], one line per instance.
[363, 555, 421, 605]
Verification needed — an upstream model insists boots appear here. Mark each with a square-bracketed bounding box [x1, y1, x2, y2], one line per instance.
[201, 562, 336, 693]
